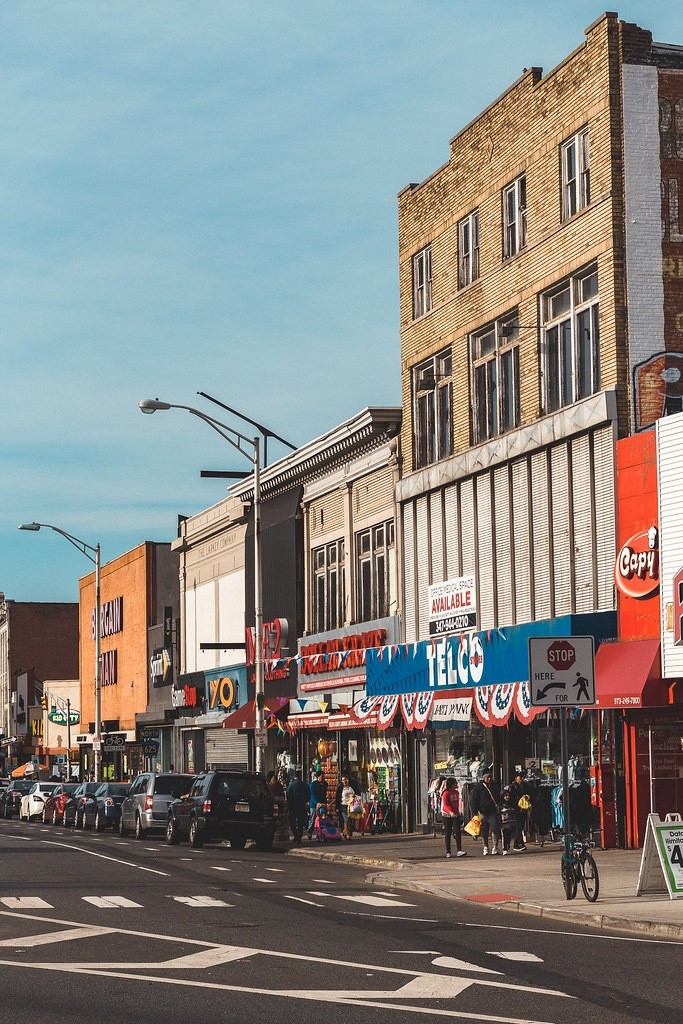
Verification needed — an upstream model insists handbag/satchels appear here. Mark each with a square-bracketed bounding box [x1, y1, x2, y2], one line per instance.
[346, 795, 364, 819]
[517, 795, 531, 810]
[463, 816, 481, 836]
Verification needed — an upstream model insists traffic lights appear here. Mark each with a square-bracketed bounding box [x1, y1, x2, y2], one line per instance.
[41, 695, 50, 710]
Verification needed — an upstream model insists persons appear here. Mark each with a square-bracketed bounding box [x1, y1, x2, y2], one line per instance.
[90, 771, 95, 782]
[319, 808, 336, 833]
[442, 779, 466, 857]
[268, 774, 285, 800]
[286, 773, 310, 842]
[307, 771, 328, 839]
[472, 768, 533, 854]
[335, 776, 359, 839]
[168, 765, 179, 774]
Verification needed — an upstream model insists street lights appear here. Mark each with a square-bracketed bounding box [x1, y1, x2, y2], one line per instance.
[16, 521, 102, 783]
[137, 397, 265, 777]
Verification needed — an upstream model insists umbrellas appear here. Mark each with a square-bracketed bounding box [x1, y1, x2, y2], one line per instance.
[12, 761, 49, 777]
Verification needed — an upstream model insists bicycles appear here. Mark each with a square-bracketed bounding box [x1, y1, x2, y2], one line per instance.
[561, 827, 600, 902]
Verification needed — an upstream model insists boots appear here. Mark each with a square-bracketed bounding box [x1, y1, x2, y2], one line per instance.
[344, 823, 349, 839]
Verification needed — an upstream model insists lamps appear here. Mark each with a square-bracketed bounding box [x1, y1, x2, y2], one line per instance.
[420, 373, 452, 389]
[495, 326, 542, 335]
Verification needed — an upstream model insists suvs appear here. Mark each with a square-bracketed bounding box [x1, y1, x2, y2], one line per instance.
[118, 772, 197, 841]
[165, 769, 279, 846]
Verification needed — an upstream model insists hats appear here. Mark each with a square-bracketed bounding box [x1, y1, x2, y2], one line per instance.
[513, 771, 525, 778]
[482, 767, 493, 779]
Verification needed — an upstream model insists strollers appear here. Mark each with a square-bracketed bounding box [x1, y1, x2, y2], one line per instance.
[315, 807, 342, 842]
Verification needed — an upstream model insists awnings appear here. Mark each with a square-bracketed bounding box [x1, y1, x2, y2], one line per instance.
[582, 639, 671, 710]
[327, 704, 381, 730]
[221, 695, 297, 728]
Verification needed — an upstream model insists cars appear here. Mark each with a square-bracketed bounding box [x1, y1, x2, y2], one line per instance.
[62, 784, 103, 829]
[18, 782, 63, 822]
[41, 785, 80, 825]
[0, 778, 42, 819]
[83, 783, 134, 834]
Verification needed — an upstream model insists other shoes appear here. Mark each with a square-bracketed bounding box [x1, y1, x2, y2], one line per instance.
[446, 853, 452, 858]
[297, 838, 301, 844]
[503, 850, 510, 855]
[308, 831, 312, 840]
[483, 847, 488, 855]
[457, 851, 467, 856]
[513, 846, 525, 851]
[492, 848, 498, 854]
